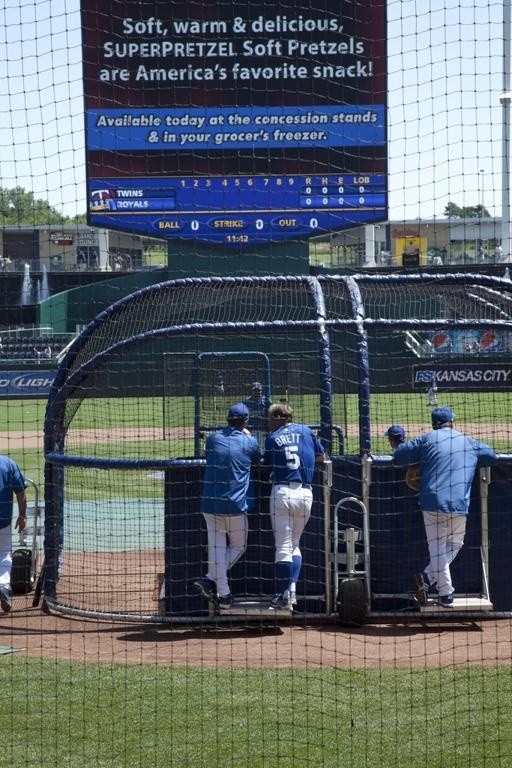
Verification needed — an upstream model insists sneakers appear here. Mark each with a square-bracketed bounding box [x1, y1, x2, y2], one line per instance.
[412, 570, 430, 606]
[218, 593, 234, 609]
[270, 591, 298, 611]
[435, 591, 455, 607]
[0, 586, 12, 612]
[194, 576, 217, 600]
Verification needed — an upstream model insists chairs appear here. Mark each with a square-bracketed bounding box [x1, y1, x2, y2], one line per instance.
[0, 335, 81, 366]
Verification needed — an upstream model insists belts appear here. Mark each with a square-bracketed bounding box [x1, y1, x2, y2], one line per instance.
[273, 481, 311, 488]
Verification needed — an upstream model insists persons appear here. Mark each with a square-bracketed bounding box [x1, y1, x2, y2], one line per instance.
[356, 239, 505, 264]
[0, 249, 133, 271]
[0, 454, 30, 613]
[211, 367, 228, 395]
[191, 402, 261, 610]
[391, 405, 497, 609]
[425, 373, 439, 405]
[383, 422, 409, 449]
[265, 402, 327, 608]
[243, 377, 273, 459]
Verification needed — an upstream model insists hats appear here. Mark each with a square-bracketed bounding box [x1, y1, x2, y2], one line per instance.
[383, 425, 405, 439]
[249, 381, 263, 392]
[430, 406, 456, 426]
[226, 402, 252, 421]
